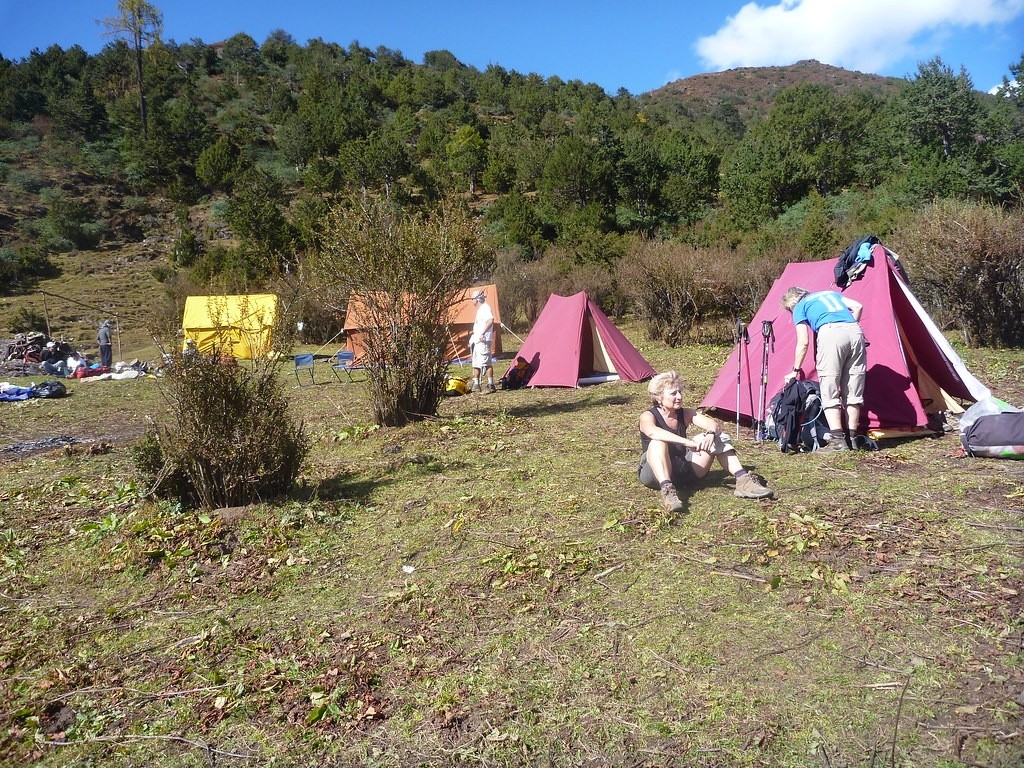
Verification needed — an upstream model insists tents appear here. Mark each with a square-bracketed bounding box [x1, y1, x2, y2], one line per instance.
[693, 246, 995, 444]
[498, 288, 658, 389]
[180, 294, 283, 360]
[343, 283, 506, 367]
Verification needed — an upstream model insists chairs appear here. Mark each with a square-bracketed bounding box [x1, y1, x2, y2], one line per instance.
[331, 350, 355, 384]
[294, 353, 315, 387]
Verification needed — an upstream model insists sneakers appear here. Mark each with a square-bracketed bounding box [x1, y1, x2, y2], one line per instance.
[482, 384, 497, 394]
[851, 432, 880, 451]
[734, 473, 774, 498]
[925, 412, 952, 433]
[661, 481, 684, 512]
[471, 379, 481, 392]
[816, 435, 849, 453]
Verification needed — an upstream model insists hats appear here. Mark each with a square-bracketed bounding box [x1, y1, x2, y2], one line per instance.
[46, 342, 56, 348]
[186, 339, 194, 344]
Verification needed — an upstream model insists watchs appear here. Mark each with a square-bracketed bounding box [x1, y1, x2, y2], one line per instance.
[703, 430, 716, 440]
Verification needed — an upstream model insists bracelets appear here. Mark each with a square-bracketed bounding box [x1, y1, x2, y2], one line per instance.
[481, 333, 485, 335]
[792, 367, 802, 372]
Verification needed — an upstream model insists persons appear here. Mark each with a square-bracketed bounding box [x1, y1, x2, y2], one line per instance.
[182, 339, 199, 358]
[95, 320, 112, 367]
[781, 286, 867, 454]
[65, 350, 87, 379]
[467, 290, 495, 394]
[41, 342, 66, 376]
[85, 354, 110, 373]
[636, 369, 774, 512]
